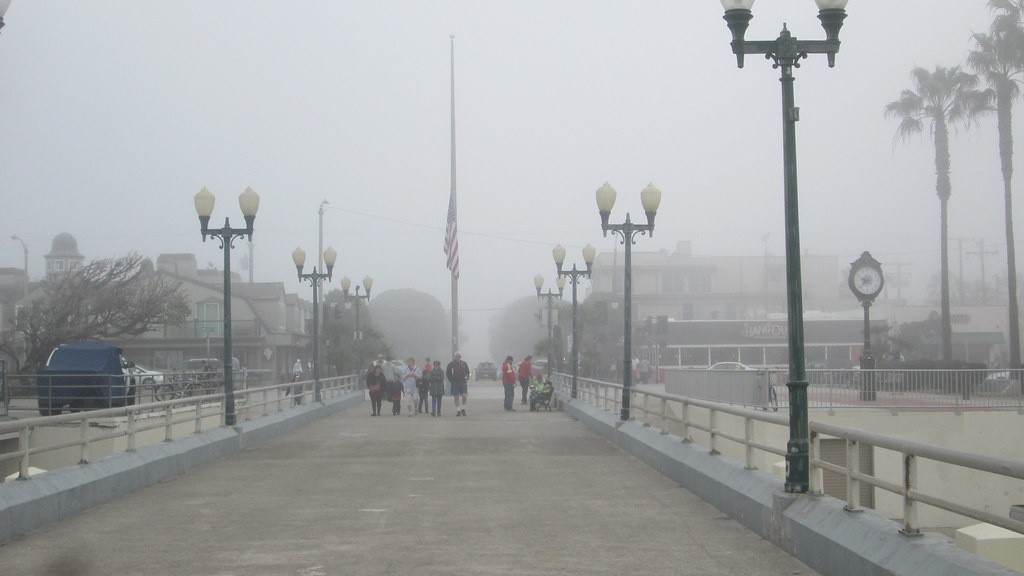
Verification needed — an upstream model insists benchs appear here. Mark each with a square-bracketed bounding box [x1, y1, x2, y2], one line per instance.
[531, 387, 554, 413]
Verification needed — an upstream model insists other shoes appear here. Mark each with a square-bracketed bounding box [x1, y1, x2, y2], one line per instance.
[397, 411, 400, 416]
[418, 409, 422, 412]
[393, 410, 396, 415]
[431, 413, 436, 417]
[461, 409, 466, 416]
[377, 413, 381, 416]
[370, 413, 376, 416]
[456, 411, 461, 416]
[408, 412, 412, 416]
[425, 410, 429, 413]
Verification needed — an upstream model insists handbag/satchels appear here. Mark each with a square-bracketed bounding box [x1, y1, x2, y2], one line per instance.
[370, 383, 381, 392]
[416, 379, 424, 387]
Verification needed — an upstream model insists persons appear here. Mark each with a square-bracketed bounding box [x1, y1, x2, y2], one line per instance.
[425, 357, 432, 371]
[429, 361, 444, 417]
[503, 356, 517, 411]
[232, 353, 240, 370]
[632, 355, 652, 383]
[285, 375, 305, 406]
[416, 370, 431, 414]
[293, 359, 303, 376]
[385, 372, 405, 416]
[399, 357, 423, 417]
[530, 381, 554, 411]
[446, 351, 469, 416]
[367, 353, 406, 416]
[518, 356, 532, 404]
[201, 359, 216, 378]
[530, 375, 545, 395]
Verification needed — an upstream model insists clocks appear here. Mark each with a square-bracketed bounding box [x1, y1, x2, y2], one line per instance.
[612, 301, 619, 310]
[850, 252, 884, 304]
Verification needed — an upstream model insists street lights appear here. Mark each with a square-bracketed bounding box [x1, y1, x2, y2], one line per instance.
[340, 275, 372, 375]
[595, 180, 662, 418]
[292, 246, 336, 401]
[720, 0, 849, 494]
[553, 244, 597, 399]
[11, 236, 28, 273]
[534, 273, 566, 382]
[194, 185, 259, 426]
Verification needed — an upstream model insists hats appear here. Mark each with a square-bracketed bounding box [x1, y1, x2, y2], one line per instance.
[546, 381, 552, 385]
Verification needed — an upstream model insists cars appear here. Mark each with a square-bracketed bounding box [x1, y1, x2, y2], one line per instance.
[390, 359, 409, 382]
[129, 365, 163, 391]
[705, 362, 758, 371]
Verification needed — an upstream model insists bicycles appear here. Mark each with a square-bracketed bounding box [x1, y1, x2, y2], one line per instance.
[154, 369, 211, 402]
[761, 366, 779, 412]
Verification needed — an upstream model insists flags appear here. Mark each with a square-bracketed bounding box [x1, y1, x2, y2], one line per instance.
[443, 191, 459, 279]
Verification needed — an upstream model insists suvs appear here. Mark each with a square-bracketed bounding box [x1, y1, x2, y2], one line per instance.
[37, 344, 136, 417]
[474, 361, 498, 382]
